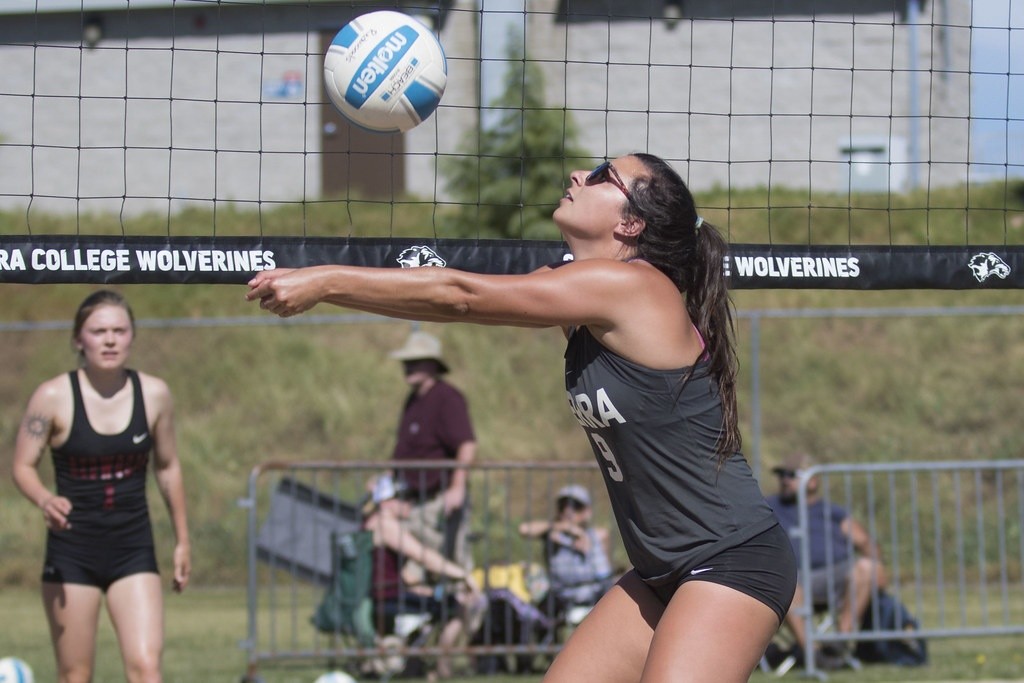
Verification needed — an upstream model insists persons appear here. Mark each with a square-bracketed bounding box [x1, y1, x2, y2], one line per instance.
[245, 151, 799, 683]
[517, 483, 617, 648]
[10, 289, 194, 681]
[762, 452, 893, 670]
[389, 329, 481, 604]
[359, 477, 490, 682]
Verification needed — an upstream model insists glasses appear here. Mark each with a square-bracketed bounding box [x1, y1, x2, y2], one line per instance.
[586, 161, 645, 217]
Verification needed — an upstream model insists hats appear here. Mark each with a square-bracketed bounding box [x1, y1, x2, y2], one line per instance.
[387, 331, 449, 373]
[555, 483, 591, 508]
[770, 452, 818, 475]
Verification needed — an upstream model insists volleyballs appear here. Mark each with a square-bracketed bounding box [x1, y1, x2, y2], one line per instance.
[324, 11, 447, 136]
[1, 655, 33, 683]
